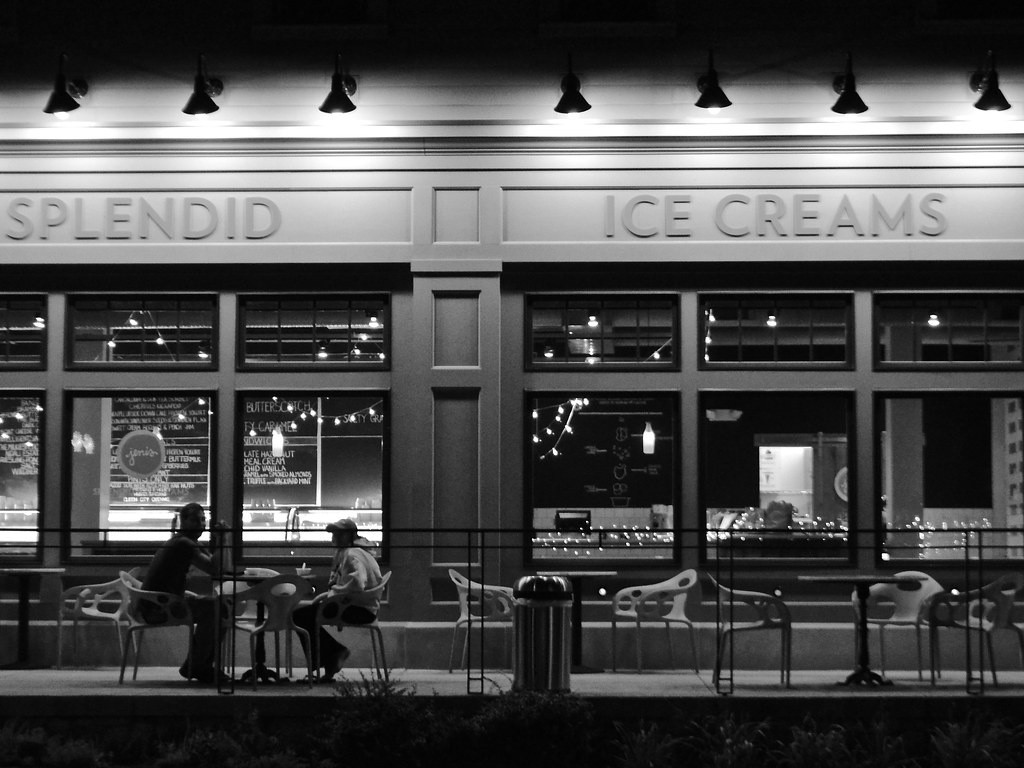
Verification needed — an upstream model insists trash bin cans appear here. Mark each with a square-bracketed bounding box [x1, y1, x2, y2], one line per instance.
[513, 575, 574, 696]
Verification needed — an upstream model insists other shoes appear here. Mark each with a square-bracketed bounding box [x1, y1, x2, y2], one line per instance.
[179, 658, 229, 686]
[320, 646, 351, 683]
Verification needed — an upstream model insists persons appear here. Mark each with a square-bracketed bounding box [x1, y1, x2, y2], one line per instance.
[138, 503, 230, 683]
[292, 519, 384, 679]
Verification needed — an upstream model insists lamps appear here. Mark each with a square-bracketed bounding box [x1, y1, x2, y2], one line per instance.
[967, 48, 1011, 111]
[41, 52, 89, 114]
[552, 53, 592, 113]
[183, 52, 226, 116]
[319, 54, 357, 115]
[318, 337, 332, 350]
[831, 53, 870, 115]
[695, 47, 733, 109]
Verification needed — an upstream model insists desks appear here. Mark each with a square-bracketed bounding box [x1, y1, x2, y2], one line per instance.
[0, 568, 66, 670]
[536, 571, 619, 677]
[223, 572, 317, 686]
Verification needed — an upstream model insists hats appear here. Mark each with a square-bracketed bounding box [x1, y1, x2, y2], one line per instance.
[325, 518, 358, 533]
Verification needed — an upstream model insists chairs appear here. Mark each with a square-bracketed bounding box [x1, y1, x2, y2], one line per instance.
[218, 572, 314, 690]
[49, 567, 141, 668]
[613, 569, 699, 675]
[710, 573, 793, 685]
[448, 567, 518, 674]
[214, 568, 293, 678]
[851, 571, 944, 689]
[317, 570, 393, 685]
[799, 576, 929, 686]
[115, 570, 200, 686]
[926, 571, 1024, 686]
